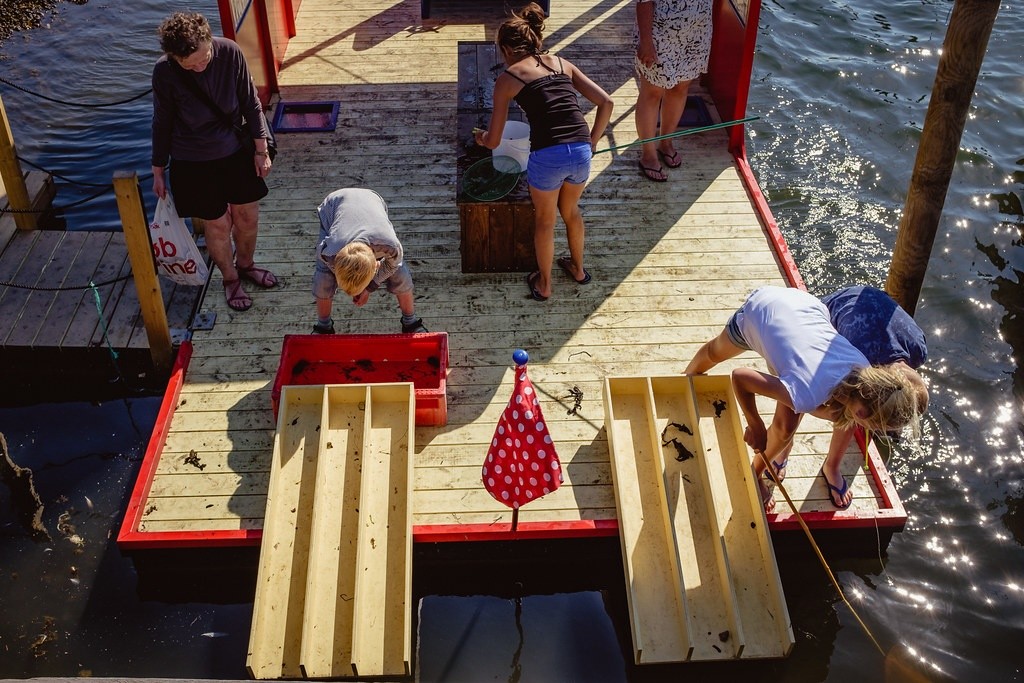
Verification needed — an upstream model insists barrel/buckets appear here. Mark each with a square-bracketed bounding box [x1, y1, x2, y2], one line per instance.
[492, 121, 531, 173]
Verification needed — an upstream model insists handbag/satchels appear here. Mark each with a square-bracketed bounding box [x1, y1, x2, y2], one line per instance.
[148, 190, 210, 286]
[240, 117, 277, 175]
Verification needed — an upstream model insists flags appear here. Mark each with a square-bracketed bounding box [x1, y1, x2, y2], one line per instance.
[482, 364, 564, 510]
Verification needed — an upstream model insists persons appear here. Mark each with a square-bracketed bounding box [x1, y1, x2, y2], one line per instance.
[311, 189, 428, 334]
[763, 287, 929, 511]
[635, 0, 713, 183]
[471, 3, 614, 300]
[151, 11, 279, 311]
[683, 286, 917, 514]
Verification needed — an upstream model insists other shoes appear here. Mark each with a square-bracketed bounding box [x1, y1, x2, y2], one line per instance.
[310, 318, 335, 335]
[400, 316, 429, 334]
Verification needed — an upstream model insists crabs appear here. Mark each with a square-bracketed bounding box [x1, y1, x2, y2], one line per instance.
[64, 533, 86, 548]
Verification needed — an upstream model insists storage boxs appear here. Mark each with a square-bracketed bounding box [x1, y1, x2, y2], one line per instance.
[272, 331, 451, 428]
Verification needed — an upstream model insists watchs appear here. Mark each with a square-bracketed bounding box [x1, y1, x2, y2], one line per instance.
[255, 151, 270, 157]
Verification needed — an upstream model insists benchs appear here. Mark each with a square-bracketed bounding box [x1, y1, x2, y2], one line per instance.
[456, 40, 540, 273]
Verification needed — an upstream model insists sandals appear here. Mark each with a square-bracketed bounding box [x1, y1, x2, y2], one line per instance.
[235, 261, 278, 289]
[223, 277, 252, 311]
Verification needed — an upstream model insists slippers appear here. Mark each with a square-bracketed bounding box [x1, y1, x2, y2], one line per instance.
[656, 148, 682, 168]
[527, 271, 545, 301]
[639, 159, 668, 182]
[757, 474, 774, 512]
[821, 467, 852, 508]
[556, 257, 591, 284]
[764, 460, 787, 483]
